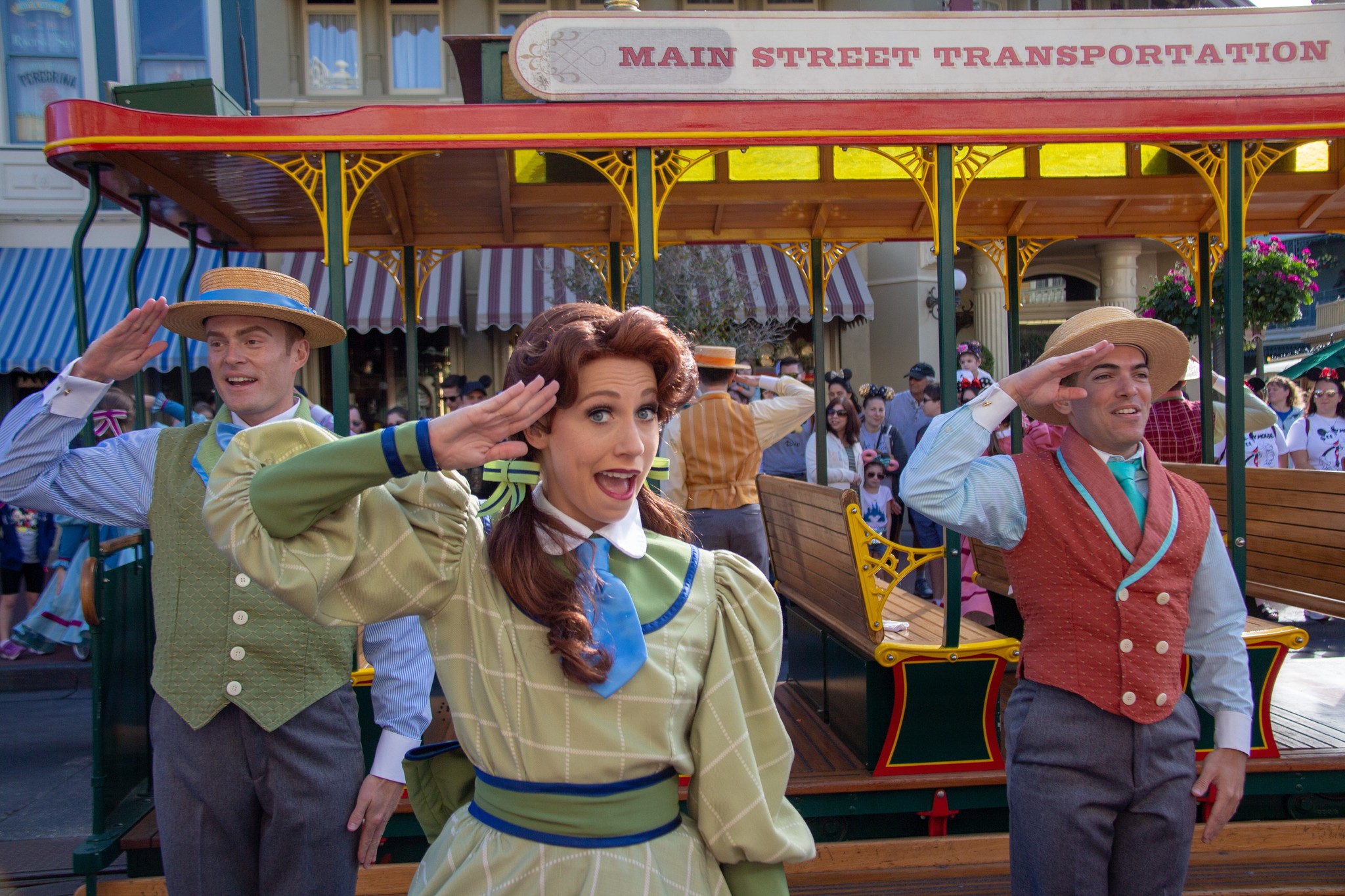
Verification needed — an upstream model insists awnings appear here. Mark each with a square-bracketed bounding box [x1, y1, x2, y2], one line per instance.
[475, 243, 874, 335]
[279, 249, 465, 334]
[1248, 337, 1345, 381]
[0, 247, 267, 376]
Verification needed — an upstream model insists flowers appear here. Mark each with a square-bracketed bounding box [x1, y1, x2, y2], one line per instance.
[1135, 237, 1320, 350]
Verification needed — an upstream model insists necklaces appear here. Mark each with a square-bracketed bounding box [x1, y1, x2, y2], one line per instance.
[1321, 416, 1338, 427]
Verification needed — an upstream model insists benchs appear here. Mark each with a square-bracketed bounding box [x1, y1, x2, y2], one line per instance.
[970, 537, 1309, 760]
[1160, 460, 1345, 622]
[752, 471, 1019, 778]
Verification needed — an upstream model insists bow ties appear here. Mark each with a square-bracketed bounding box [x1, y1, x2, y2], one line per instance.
[216, 422, 247, 452]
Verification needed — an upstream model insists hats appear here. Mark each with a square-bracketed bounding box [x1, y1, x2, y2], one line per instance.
[1018, 305, 1191, 425]
[903, 363, 935, 380]
[157, 267, 346, 349]
[691, 345, 751, 370]
[461, 381, 488, 396]
[1179, 359, 1201, 381]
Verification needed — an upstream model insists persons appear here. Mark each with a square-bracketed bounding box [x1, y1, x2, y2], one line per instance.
[203, 301, 817, 896]
[1143, 356, 1345, 620]
[0, 269, 436, 896]
[684, 339, 1065, 628]
[293, 375, 503, 499]
[657, 342, 817, 582]
[0, 388, 221, 836]
[897, 306, 1255, 896]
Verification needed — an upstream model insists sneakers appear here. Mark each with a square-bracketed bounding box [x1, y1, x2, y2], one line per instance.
[0, 639, 25, 660]
[915, 577, 934, 599]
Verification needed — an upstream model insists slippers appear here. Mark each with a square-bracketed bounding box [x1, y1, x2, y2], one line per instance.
[1257, 603, 1279, 622]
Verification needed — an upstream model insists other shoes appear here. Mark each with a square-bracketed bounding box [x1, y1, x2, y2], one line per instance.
[1303, 610, 1325, 619]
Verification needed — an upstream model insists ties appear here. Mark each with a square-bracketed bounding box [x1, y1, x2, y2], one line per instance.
[1105, 458, 1147, 533]
[558, 536, 647, 697]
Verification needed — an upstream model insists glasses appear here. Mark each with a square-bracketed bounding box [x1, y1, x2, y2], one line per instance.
[827, 408, 847, 418]
[1315, 389, 1339, 399]
[387, 420, 406, 428]
[780, 371, 804, 378]
[961, 400, 968, 404]
[440, 393, 461, 403]
[350, 420, 361, 426]
[865, 470, 886, 480]
[923, 398, 935, 403]
